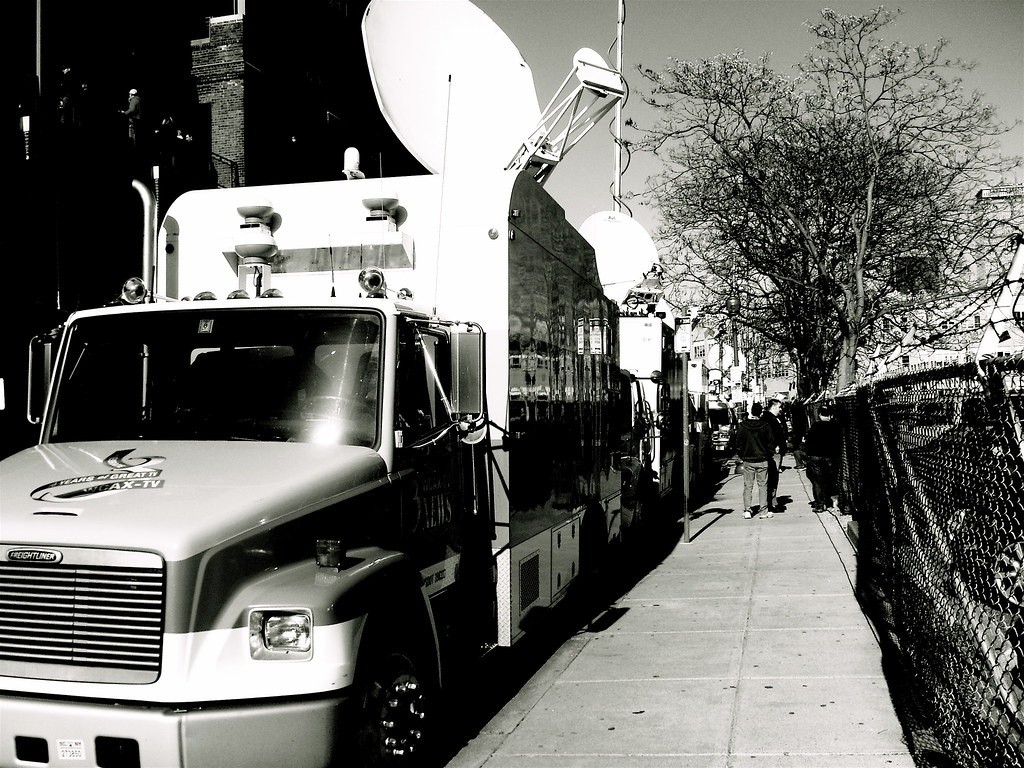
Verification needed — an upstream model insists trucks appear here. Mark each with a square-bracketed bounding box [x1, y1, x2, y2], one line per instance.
[0, 44, 681, 768]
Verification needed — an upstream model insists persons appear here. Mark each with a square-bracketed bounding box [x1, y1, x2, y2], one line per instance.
[121, 88, 142, 146]
[760, 399, 789, 513]
[793, 429, 810, 469]
[804, 405, 840, 512]
[735, 403, 773, 519]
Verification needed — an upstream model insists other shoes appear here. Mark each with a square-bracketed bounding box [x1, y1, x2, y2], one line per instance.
[743, 509, 753, 518]
[811, 502, 826, 513]
[767, 504, 786, 512]
[759, 511, 774, 519]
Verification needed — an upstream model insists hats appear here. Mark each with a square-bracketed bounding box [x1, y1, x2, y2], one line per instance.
[819, 405, 832, 416]
